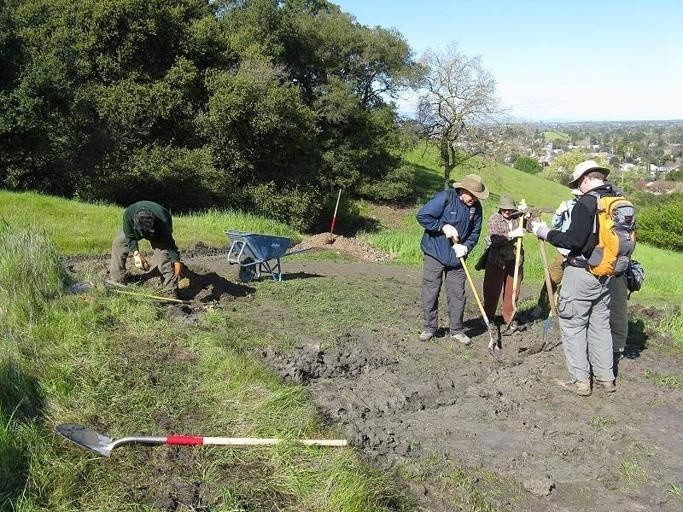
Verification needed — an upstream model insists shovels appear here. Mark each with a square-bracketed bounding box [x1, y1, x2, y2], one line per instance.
[326, 189, 342, 244]
[536, 216, 562, 352]
[54, 422, 349, 458]
[502, 198, 525, 333]
[70, 283, 194, 304]
[452, 237, 500, 359]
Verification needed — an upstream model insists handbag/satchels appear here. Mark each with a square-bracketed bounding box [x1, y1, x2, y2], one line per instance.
[628, 258, 645, 292]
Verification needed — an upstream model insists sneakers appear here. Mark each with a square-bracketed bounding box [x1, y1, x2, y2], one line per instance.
[563, 379, 591, 396]
[613, 351, 624, 363]
[507, 321, 526, 331]
[419, 331, 433, 340]
[450, 331, 472, 345]
[481, 319, 497, 329]
[591, 375, 616, 393]
[531, 306, 542, 318]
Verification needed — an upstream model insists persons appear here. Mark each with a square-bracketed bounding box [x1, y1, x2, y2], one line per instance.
[530, 159, 616, 397]
[474, 196, 535, 331]
[529, 186, 585, 318]
[414, 173, 489, 345]
[609, 272, 627, 354]
[109, 199, 183, 300]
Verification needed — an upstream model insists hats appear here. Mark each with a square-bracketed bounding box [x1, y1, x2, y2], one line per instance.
[453, 174, 489, 200]
[496, 195, 516, 209]
[568, 160, 610, 195]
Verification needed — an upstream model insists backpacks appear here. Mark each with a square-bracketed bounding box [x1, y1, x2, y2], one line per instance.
[586, 192, 637, 275]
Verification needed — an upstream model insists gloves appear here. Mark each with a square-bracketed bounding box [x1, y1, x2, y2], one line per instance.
[172, 262, 184, 283]
[531, 221, 549, 240]
[556, 201, 569, 215]
[442, 224, 459, 241]
[453, 244, 468, 258]
[517, 202, 530, 219]
[135, 252, 150, 271]
[508, 227, 526, 239]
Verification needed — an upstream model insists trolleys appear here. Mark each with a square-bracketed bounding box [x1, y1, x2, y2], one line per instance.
[225, 229, 313, 283]
